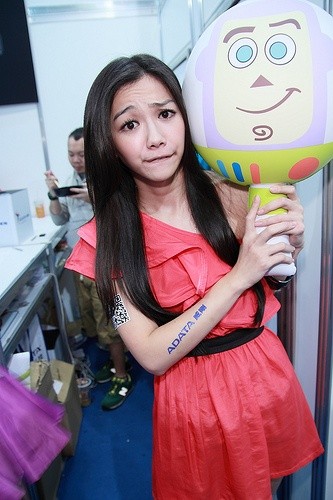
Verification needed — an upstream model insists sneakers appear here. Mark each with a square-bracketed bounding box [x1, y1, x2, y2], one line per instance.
[100, 374, 135, 409]
[94, 355, 134, 384]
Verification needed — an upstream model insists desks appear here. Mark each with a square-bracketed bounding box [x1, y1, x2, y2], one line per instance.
[0, 216, 73, 371]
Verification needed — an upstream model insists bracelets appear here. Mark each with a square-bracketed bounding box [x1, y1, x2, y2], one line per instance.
[264, 275, 294, 291]
[47, 192, 58, 200]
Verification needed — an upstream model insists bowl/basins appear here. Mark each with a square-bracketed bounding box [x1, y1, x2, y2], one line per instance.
[54, 185, 84, 197]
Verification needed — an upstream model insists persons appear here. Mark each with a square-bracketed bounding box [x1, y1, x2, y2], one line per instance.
[44, 128, 134, 412]
[64, 54, 325, 500]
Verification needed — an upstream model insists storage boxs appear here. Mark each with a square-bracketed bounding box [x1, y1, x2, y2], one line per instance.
[6, 359, 83, 500]
[1, 188, 35, 247]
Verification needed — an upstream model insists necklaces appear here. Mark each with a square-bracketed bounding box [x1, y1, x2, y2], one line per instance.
[194, 225, 197, 234]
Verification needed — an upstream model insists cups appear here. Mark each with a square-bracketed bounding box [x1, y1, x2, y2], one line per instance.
[76, 378, 92, 407]
[33, 200, 45, 218]
